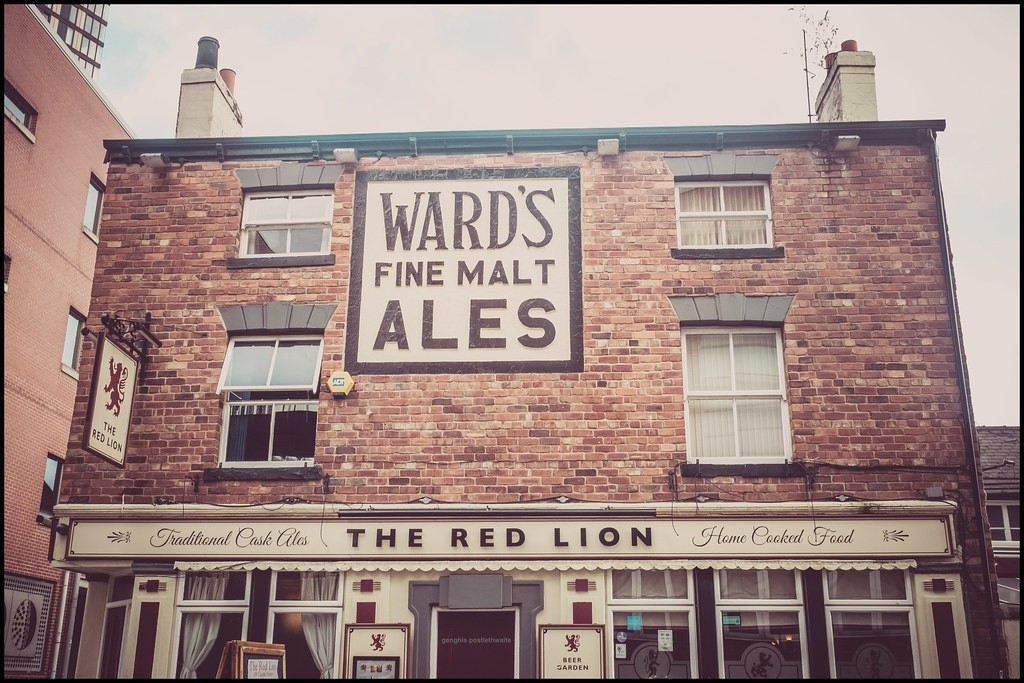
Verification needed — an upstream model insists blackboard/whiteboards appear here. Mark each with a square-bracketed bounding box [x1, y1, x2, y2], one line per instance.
[213, 641, 286, 680]
